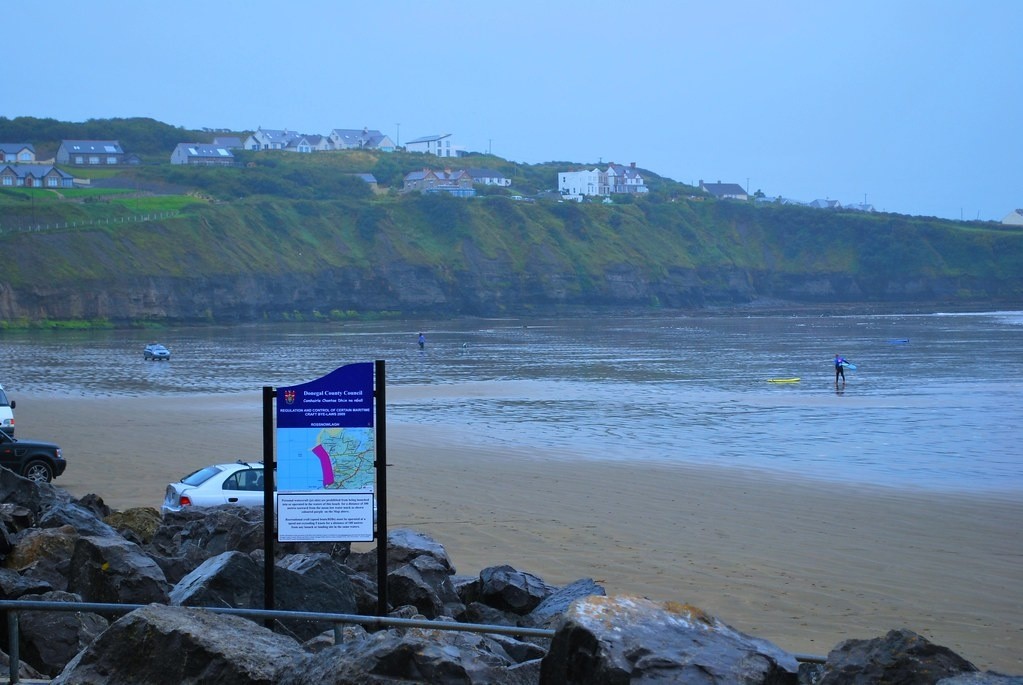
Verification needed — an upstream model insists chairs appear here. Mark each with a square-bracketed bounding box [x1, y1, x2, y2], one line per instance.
[246, 472, 257, 490]
[257, 476, 263, 491]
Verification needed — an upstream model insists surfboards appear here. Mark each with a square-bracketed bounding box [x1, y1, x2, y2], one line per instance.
[766, 378, 801, 382]
[841, 363, 857, 370]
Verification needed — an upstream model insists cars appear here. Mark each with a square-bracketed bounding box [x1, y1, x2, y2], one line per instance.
[143, 342, 171, 361]
[160, 459, 277, 522]
[0, 430, 68, 483]
[0, 384, 15, 442]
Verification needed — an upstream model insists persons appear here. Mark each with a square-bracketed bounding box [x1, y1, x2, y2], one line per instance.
[417, 333, 426, 348]
[834, 354, 850, 385]
